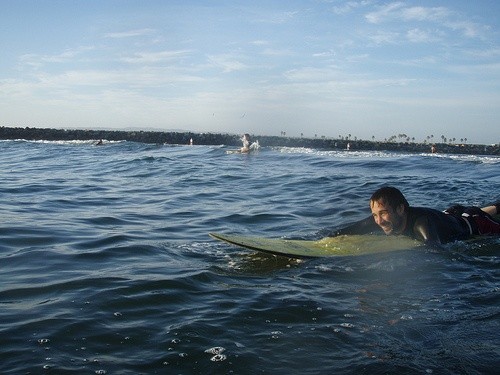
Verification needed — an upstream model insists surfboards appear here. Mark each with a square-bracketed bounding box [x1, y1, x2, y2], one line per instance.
[206, 233, 499, 258]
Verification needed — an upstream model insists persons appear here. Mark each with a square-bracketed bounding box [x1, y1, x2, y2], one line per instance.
[241, 134, 251, 152]
[329, 186, 500, 252]
[430, 145, 436, 154]
